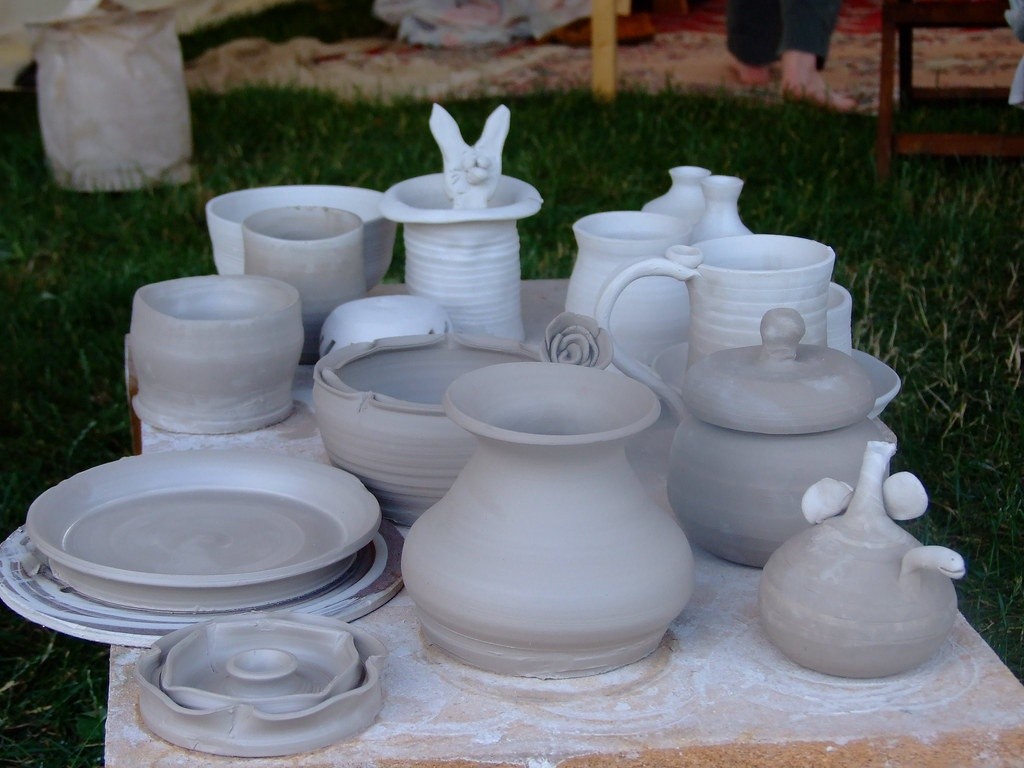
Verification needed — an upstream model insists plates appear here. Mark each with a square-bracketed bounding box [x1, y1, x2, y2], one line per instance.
[0, 502, 408, 649]
[28, 450, 383, 613]
[856, 354, 901, 413]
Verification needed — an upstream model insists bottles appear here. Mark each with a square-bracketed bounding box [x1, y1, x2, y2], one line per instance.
[642, 166, 750, 245]
[666, 312, 966, 681]
[565, 208, 692, 365]
[401, 361, 695, 680]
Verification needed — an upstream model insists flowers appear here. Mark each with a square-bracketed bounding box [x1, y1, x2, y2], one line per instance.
[539, 312, 613, 371]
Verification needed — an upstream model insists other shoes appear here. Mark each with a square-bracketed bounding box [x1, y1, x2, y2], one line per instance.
[551, 15, 655, 48]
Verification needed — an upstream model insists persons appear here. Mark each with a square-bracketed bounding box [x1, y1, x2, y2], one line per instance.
[723, 0, 861, 115]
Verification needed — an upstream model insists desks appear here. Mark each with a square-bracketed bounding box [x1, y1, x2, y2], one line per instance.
[104, 279, 1024, 767]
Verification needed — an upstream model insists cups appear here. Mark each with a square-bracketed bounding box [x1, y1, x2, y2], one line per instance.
[593, 234, 837, 415]
[830, 282, 856, 355]
[129, 275, 305, 436]
[382, 170, 552, 343]
[242, 204, 367, 364]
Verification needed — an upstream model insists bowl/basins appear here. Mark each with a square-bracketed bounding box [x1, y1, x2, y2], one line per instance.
[314, 333, 542, 526]
[206, 184, 397, 302]
